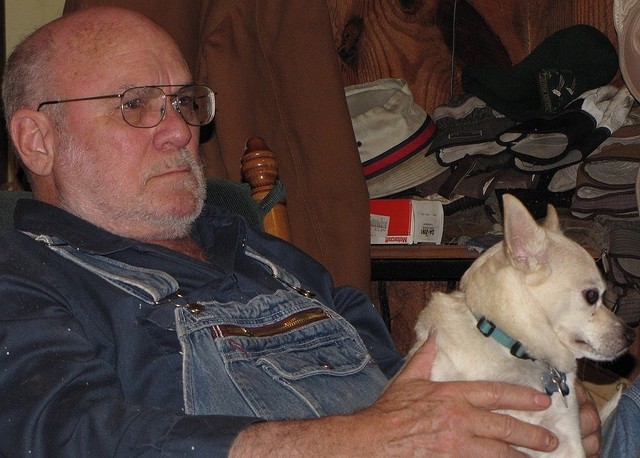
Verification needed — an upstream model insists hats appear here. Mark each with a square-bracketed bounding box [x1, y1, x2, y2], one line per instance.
[344, 76, 449, 202]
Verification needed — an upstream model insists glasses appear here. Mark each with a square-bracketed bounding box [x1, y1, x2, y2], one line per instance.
[36, 83, 217, 129]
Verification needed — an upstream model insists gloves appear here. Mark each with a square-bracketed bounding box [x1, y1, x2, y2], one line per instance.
[573, 238, 640, 384]
[424, 85, 640, 239]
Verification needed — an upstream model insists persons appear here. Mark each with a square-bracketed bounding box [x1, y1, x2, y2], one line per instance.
[0, 4, 639, 453]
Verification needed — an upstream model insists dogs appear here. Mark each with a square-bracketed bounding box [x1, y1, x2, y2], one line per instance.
[375, 193, 635, 458]
